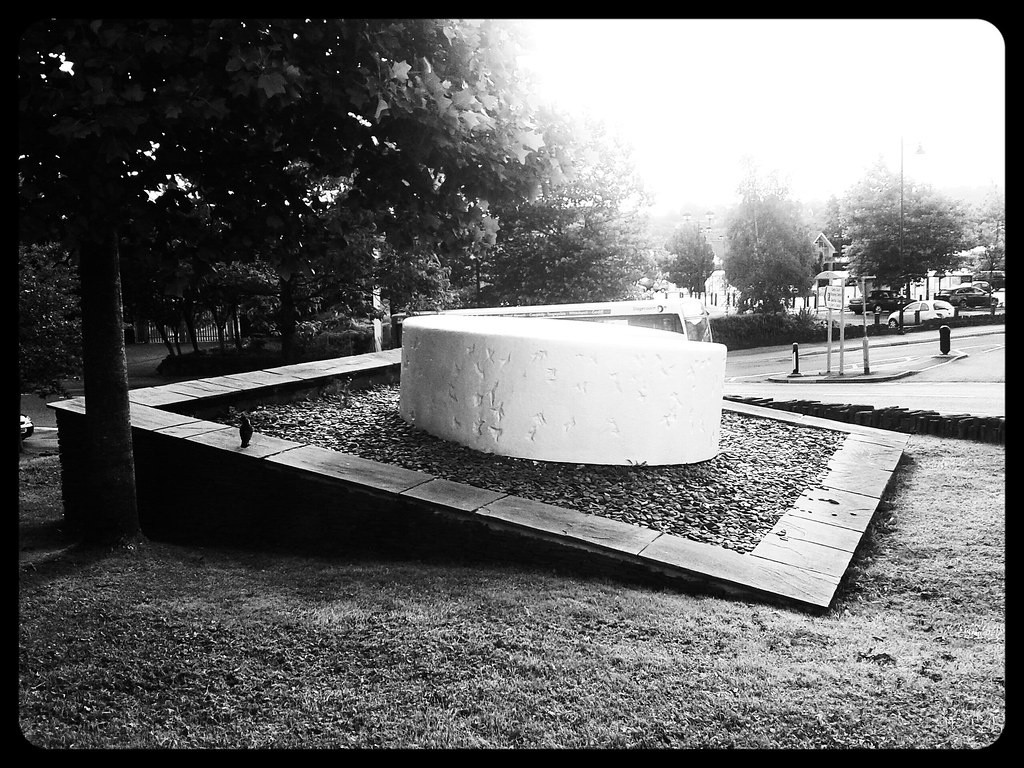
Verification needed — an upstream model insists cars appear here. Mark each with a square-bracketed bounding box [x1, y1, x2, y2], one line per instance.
[20, 414, 35, 439]
[934, 285, 999, 309]
[961, 281, 1005, 308]
[849, 289, 918, 314]
[886, 298, 955, 329]
